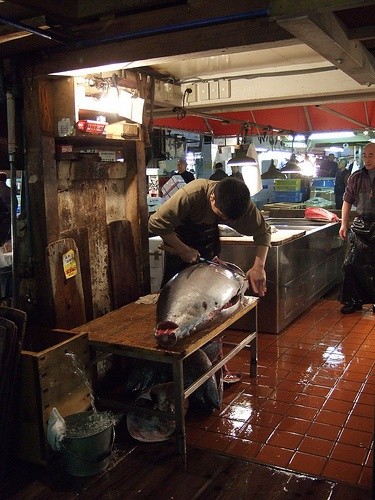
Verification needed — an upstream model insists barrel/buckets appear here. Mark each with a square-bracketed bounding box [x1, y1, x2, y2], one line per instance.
[58, 412, 116, 476]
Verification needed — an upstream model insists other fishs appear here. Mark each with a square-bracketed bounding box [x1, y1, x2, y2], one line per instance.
[153, 256, 249, 348]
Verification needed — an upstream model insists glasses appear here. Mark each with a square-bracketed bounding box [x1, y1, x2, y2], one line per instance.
[361, 155, 375, 160]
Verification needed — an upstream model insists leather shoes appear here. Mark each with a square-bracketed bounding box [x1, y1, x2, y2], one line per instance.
[341, 304, 362, 313]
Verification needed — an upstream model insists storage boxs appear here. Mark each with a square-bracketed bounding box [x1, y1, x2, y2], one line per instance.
[272, 178, 335, 203]
[161, 175, 186, 200]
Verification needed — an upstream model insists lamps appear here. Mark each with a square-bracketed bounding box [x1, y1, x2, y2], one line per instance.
[281, 137, 303, 173]
[226, 128, 256, 166]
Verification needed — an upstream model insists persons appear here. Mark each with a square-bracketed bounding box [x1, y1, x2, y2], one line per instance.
[231, 166, 244, 180]
[338, 143, 375, 317]
[147, 178, 272, 296]
[0, 172, 18, 249]
[319, 154, 338, 180]
[209, 163, 229, 182]
[169, 159, 195, 185]
[332, 159, 352, 209]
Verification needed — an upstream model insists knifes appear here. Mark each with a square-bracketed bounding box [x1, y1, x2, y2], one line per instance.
[198, 257, 224, 269]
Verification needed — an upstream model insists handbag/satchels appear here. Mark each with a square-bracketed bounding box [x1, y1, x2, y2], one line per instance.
[350, 217, 375, 239]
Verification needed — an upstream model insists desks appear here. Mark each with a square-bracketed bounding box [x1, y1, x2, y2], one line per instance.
[72, 290, 260, 454]
